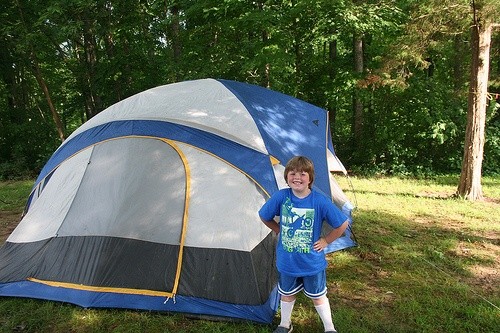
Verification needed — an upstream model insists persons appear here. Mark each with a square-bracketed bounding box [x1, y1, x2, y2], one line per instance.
[259, 154, 350, 333]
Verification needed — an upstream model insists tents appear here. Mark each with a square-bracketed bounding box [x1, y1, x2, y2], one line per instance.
[0, 76, 357, 326]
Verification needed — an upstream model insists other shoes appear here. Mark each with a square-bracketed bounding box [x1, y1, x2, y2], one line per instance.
[273, 324, 294, 333]
[323, 328, 337, 333]
